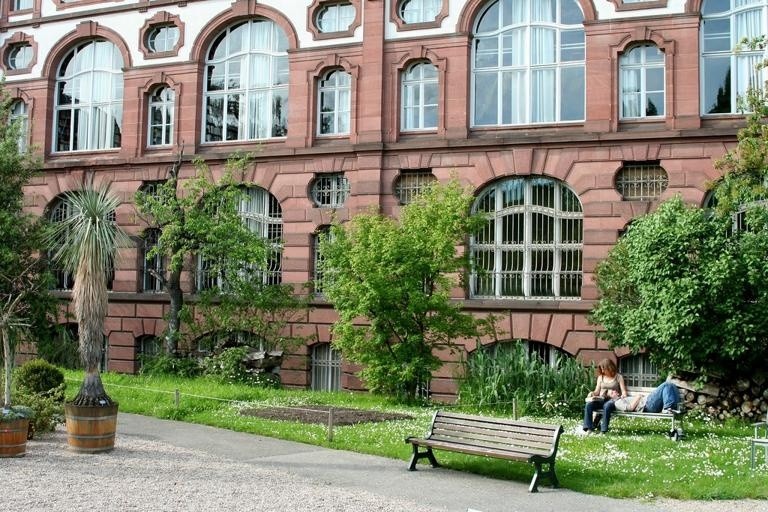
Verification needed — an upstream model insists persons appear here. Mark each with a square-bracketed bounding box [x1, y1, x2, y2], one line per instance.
[581, 357, 627, 438]
[601, 379, 681, 418]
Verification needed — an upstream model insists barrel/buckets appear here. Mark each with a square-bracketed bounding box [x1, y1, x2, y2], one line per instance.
[65, 401, 119, 454]
[0, 419, 29, 458]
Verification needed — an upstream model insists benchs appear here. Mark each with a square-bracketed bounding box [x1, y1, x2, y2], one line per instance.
[584, 385, 685, 442]
[404, 409, 564, 494]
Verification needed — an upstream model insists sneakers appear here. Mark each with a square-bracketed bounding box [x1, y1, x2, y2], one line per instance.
[665, 428, 687, 441]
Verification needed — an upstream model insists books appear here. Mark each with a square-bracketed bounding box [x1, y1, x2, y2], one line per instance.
[585, 396, 605, 402]
